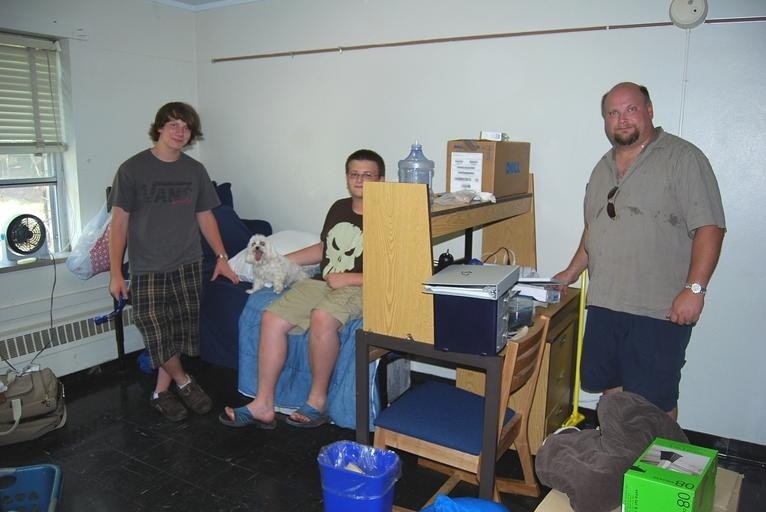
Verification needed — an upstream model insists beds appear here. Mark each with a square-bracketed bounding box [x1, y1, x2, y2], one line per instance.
[108, 185, 362, 427]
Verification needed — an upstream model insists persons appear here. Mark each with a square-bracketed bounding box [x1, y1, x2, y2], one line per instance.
[550, 82, 727, 424]
[219, 149, 386, 431]
[109, 102, 240, 422]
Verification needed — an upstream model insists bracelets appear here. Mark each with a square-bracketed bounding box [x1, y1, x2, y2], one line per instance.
[216, 253, 229, 260]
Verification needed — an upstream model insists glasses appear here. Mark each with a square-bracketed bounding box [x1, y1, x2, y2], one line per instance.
[606, 185, 619, 221]
[347, 172, 381, 180]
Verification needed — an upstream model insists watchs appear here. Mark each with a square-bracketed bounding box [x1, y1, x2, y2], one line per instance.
[682, 282, 707, 296]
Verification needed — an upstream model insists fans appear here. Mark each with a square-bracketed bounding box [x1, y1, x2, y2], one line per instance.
[5, 214, 47, 261]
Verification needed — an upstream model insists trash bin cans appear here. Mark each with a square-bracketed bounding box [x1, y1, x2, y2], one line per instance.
[318, 440, 401, 512]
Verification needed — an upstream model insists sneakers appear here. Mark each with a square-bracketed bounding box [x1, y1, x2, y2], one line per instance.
[148, 389, 188, 423]
[175, 373, 214, 416]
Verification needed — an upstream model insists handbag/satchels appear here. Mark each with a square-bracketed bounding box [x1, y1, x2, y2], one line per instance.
[0, 366, 68, 448]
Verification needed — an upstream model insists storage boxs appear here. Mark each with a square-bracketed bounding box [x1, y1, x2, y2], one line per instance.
[443, 135, 531, 199]
[619, 431, 717, 510]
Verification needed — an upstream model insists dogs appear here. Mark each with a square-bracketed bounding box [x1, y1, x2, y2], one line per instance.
[245, 233, 311, 294]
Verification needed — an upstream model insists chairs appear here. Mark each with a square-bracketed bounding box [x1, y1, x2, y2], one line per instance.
[371, 317, 547, 499]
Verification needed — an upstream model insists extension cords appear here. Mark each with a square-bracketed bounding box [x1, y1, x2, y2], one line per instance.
[483, 263, 531, 277]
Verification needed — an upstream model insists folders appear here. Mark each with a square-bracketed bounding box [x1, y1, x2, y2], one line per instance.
[421, 263, 520, 302]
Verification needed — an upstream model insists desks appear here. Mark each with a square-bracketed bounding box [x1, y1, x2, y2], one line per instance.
[360, 263, 587, 503]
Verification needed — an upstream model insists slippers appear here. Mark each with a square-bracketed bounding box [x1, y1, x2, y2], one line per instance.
[218, 405, 278, 430]
[285, 403, 330, 429]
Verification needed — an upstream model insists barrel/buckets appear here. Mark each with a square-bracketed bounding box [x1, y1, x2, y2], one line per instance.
[397, 138, 436, 207]
